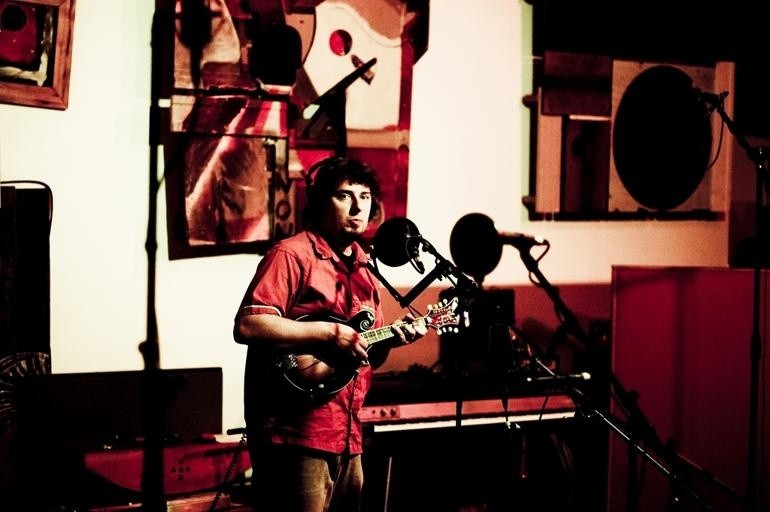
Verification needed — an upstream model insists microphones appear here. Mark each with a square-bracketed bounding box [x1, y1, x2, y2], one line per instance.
[612, 66, 712, 210]
[373, 217, 425, 273]
[450, 213, 548, 276]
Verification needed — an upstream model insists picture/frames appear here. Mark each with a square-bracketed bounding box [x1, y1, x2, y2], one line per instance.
[0, 0, 77, 111]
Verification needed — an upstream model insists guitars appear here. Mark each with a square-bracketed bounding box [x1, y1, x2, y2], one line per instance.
[275, 300, 468, 394]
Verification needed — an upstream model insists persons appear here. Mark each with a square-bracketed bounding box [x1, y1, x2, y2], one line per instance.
[232, 154, 432, 512]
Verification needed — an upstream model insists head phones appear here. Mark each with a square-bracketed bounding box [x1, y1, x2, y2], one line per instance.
[304, 161, 376, 223]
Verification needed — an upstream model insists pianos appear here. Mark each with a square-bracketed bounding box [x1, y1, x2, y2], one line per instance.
[354, 394, 577, 437]
